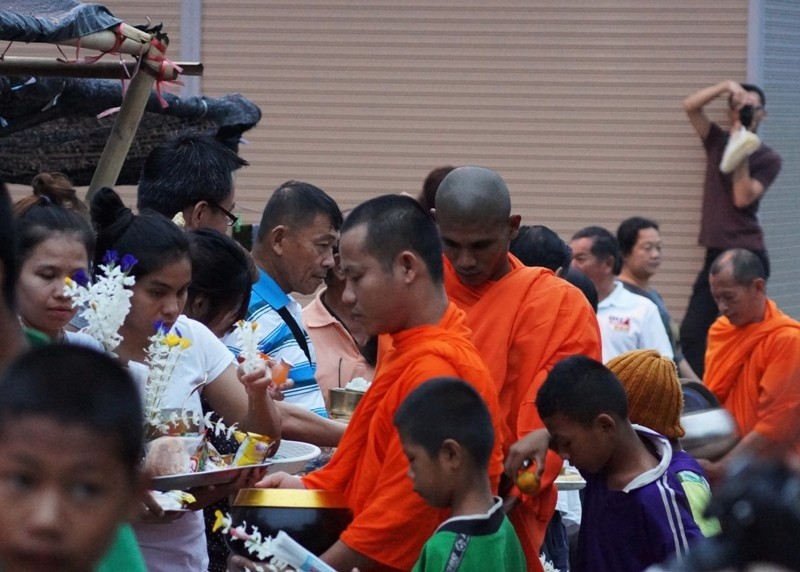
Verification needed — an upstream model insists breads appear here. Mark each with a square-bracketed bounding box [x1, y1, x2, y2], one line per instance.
[145, 436, 189, 477]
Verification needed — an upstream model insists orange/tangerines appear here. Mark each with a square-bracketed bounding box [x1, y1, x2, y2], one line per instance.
[517, 472, 540, 494]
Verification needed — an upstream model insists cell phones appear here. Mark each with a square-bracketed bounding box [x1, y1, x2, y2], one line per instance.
[739, 104, 753, 129]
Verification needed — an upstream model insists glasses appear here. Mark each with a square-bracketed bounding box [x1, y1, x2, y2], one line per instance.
[208, 196, 239, 226]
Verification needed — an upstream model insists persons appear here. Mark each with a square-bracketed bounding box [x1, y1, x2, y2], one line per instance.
[441, 164, 602, 555]
[512, 224, 598, 314]
[419, 167, 455, 213]
[12, 172, 269, 524]
[505, 355, 705, 572]
[91, 187, 281, 572]
[136, 129, 347, 533]
[570, 225, 674, 365]
[613, 216, 697, 378]
[228, 194, 498, 572]
[0, 340, 144, 571]
[0, 181, 148, 572]
[606, 350, 722, 536]
[679, 82, 783, 381]
[301, 209, 380, 413]
[698, 248, 800, 477]
[393, 376, 528, 572]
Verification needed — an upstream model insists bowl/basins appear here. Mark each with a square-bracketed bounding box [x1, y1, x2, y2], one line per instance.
[265, 440, 322, 476]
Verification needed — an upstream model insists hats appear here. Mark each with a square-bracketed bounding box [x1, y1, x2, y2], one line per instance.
[602, 349, 686, 440]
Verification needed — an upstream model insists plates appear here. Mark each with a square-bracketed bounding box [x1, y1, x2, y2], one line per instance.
[151, 463, 273, 491]
